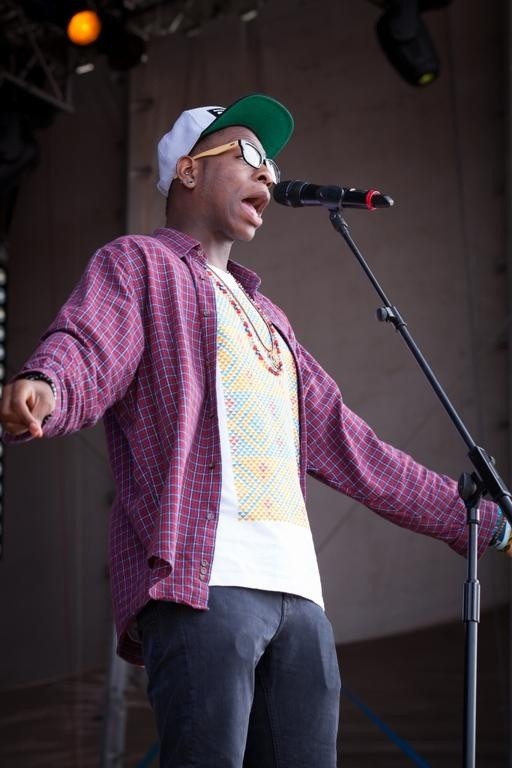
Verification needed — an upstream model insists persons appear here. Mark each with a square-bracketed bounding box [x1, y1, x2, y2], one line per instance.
[1, 94, 511, 768]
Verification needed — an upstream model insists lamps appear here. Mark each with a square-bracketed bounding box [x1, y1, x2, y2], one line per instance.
[45, 0, 146, 72]
[370, 0, 457, 87]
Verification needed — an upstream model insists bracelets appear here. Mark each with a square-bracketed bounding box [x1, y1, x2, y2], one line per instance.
[488, 506, 506, 547]
[30, 374, 57, 401]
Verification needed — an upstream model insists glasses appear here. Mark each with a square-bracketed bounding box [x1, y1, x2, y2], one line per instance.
[174, 139, 281, 185]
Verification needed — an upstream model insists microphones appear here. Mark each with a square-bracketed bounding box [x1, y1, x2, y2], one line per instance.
[272, 179, 395, 212]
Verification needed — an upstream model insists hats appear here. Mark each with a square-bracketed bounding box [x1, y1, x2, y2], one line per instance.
[156, 94, 294, 198]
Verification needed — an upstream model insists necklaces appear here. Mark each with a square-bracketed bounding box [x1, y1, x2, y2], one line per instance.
[206, 267, 285, 380]
[205, 263, 275, 354]
